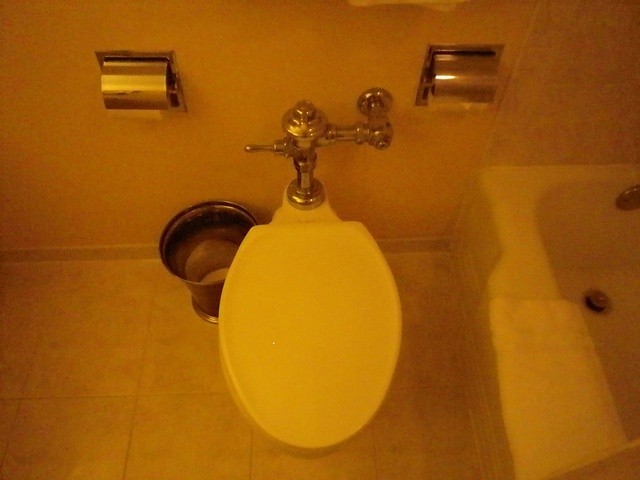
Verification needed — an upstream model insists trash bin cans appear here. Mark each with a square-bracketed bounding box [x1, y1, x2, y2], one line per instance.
[159, 199, 259, 323]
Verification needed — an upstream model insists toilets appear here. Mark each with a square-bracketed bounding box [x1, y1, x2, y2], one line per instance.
[217, 92, 401, 449]
